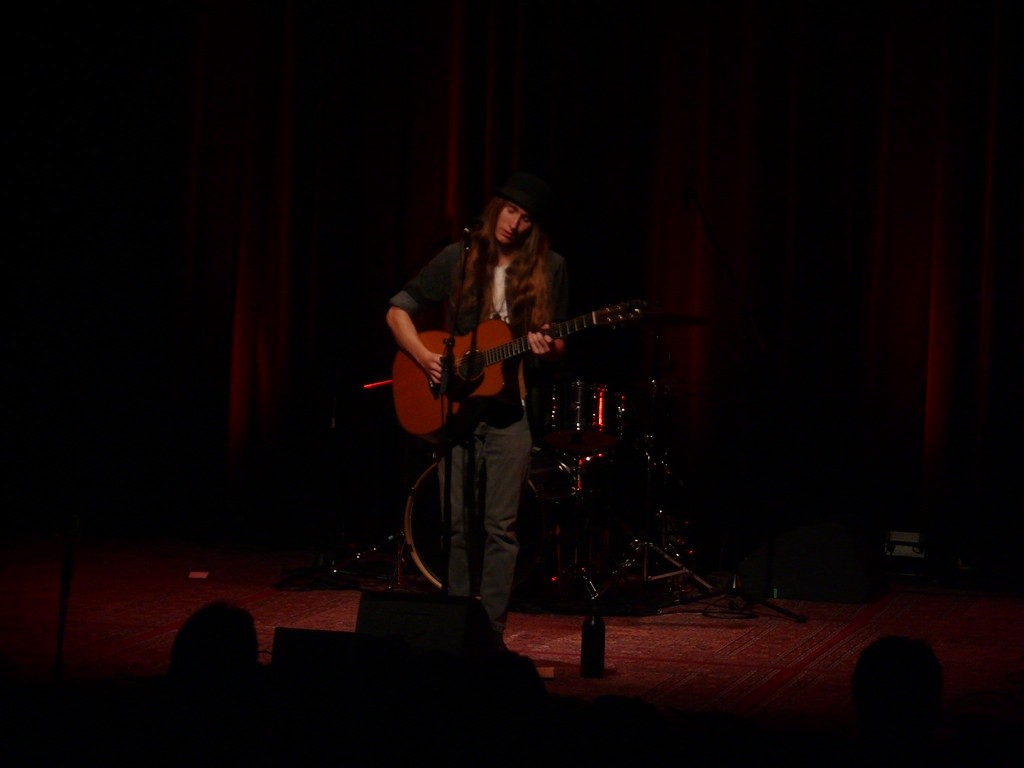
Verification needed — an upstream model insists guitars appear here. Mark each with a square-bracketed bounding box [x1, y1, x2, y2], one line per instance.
[386, 295, 649, 441]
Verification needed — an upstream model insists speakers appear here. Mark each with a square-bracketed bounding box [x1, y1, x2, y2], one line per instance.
[270, 588, 544, 702]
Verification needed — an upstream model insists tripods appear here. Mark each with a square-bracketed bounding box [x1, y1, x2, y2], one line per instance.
[589, 313, 808, 623]
[269, 386, 414, 594]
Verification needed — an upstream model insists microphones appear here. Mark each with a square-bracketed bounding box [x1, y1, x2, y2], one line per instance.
[463, 218, 483, 234]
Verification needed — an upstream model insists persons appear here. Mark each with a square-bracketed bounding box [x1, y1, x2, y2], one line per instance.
[386, 174, 566, 635]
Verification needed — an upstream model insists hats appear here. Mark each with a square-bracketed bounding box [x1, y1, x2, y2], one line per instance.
[496, 172, 547, 222]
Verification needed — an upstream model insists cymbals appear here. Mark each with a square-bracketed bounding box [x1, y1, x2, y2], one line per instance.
[601, 309, 714, 338]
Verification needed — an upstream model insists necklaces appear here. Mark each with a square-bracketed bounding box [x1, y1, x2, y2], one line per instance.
[492, 295, 507, 322]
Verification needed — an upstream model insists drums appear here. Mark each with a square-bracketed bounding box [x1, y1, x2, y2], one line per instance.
[534, 367, 651, 464]
[403, 447, 561, 597]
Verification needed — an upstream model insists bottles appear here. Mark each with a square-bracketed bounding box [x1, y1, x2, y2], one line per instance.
[580, 605, 605, 677]
